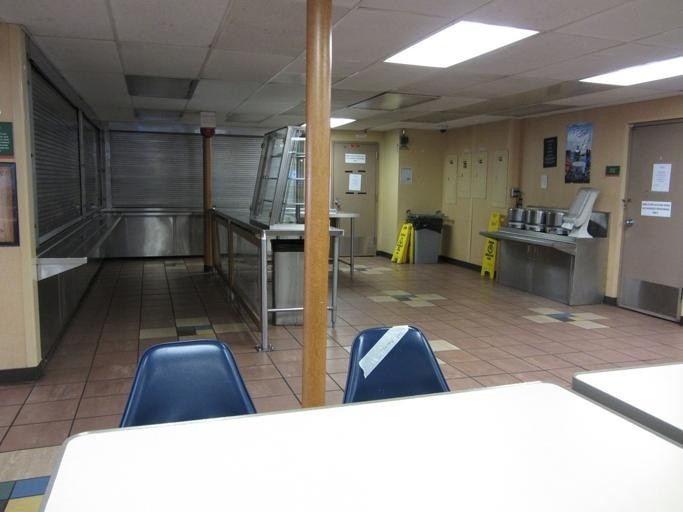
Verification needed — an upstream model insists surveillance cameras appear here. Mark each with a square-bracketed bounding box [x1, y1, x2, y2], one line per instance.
[440, 129, 447, 134]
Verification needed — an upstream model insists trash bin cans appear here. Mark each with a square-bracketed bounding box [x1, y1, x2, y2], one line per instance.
[413, 217, 443, 264]
[270, 238, 304, 326]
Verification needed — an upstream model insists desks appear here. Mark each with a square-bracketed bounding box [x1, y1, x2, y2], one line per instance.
[36, 379, 683, 512]
[212, 202, 361, 351]
[569, 361, 683, 440]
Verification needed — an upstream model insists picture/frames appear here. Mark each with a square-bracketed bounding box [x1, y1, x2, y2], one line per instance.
[0, 162, 21, 246]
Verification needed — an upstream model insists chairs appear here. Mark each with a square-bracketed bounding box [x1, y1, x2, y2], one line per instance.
[341, 326, 449, 410]
[119, 338, 257, 430]
